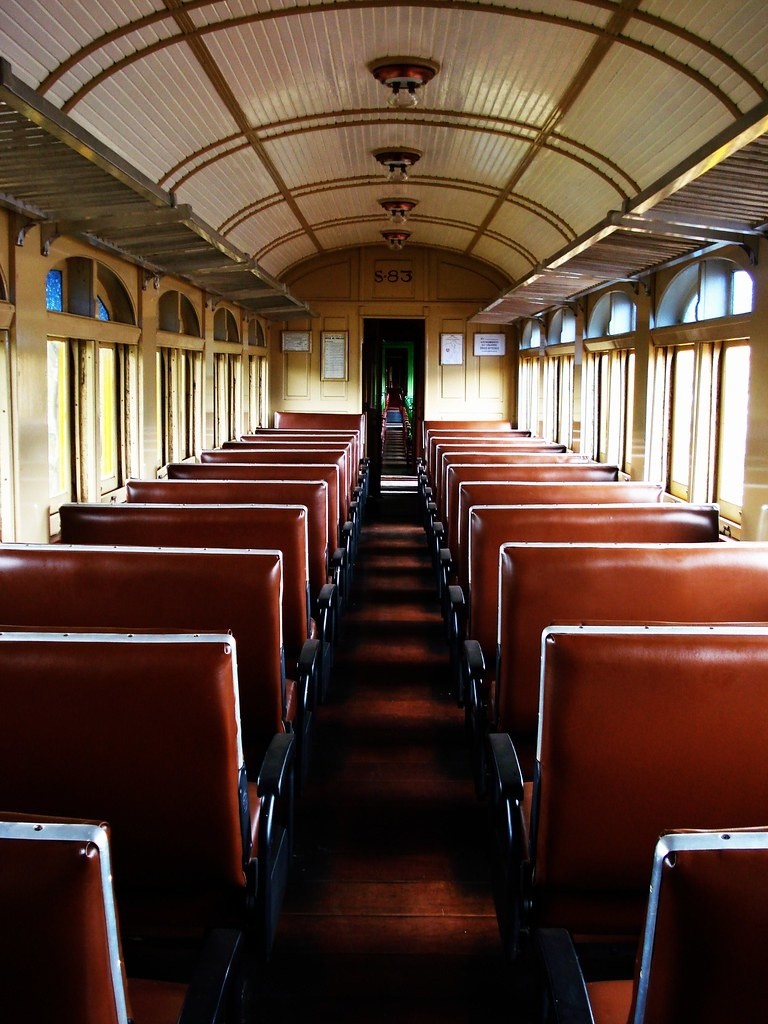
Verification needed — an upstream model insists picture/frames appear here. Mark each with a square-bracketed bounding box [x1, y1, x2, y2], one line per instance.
[320, 330, 350, 382]
[473, 331, 508, 357]
[280, 330, 313, 354]
[438, 332, 465, 367]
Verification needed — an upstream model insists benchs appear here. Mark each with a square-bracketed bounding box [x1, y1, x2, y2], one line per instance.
[1, 624, 298, 955]
[474, 622, 768, 969]
[127, 410, 664, 617]
[529, 827, 767, 1024]
[463, 541, 767, 779]
[1, 542, 321, 790]
[0, 810, 193, 1023]
[58, 502, 338, 707]
[448, 504, 718, 710]
